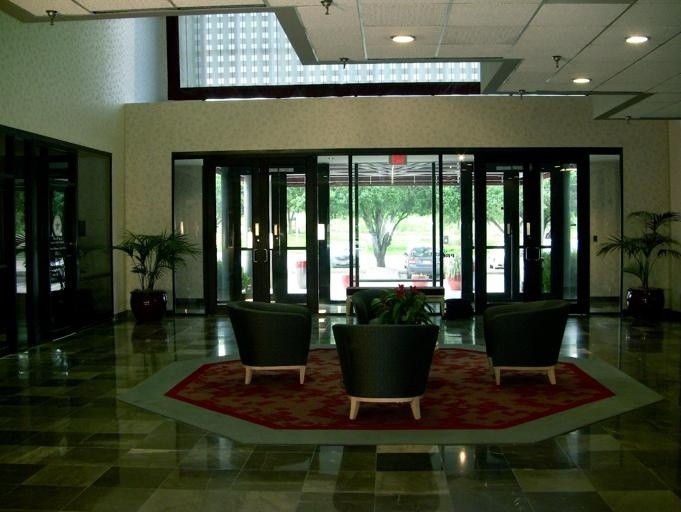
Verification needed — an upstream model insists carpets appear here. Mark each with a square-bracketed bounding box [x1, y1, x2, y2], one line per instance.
[118, 344, 664, 445]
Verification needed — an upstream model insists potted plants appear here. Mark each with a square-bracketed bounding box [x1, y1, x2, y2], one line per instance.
[593, 209, 679, 324]
[110, 228, 202, 326]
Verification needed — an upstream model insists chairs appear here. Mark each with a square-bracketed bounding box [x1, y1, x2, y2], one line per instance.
[484, 300, 572, 387]
[333, 323, 441, 422]
[353, 288, 414, 324]
[225, 301, 312, 387]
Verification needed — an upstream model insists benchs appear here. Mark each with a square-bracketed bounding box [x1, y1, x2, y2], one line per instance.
[346, 285, 445, 315]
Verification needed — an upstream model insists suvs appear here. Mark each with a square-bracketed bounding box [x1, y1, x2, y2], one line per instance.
[403, 243, 445, 282]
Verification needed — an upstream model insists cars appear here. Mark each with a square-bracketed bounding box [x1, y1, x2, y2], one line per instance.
[489, 251, 505, 270]
[330, 249, 360, 269]
